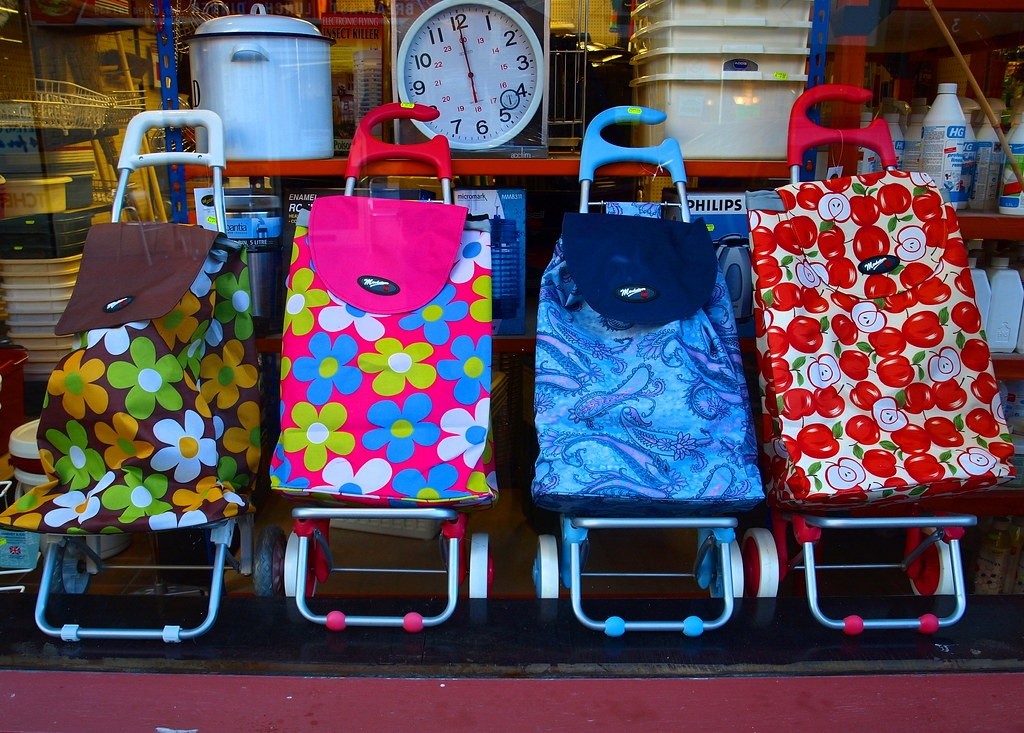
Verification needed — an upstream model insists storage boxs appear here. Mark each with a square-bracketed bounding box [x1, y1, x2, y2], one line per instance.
[451, 185, 527, 334]
[320, 10, 394, 151]
[285, 186, 437, 287]
[659, 187, 755, 338]
[628, 0, 814, 160]
[192, 187, 285, 338]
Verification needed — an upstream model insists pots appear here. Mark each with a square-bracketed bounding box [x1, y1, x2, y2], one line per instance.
[181, 4, 335, 163]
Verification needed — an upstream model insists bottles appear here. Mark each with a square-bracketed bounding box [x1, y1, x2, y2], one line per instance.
[985, 257, 1024, 353]
[857, 83, 1024, 216]
[967, 257, 991, 332]
[488, 214, 521, 321]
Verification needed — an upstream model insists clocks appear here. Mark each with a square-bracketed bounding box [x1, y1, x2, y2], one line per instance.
[398, 0, 546, 148]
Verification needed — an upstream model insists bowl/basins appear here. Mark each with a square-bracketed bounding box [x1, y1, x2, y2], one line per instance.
[0, 176, 73, 217]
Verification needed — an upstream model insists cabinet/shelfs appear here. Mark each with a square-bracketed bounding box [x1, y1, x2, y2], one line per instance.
[150, 0, 1023, 598]
[0, 0, 178, 493]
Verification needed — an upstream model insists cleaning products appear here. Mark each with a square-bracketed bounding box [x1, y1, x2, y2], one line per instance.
[861, 82, 1024, 215]
[992, 380, 1024, 490]
[965, 239, 1024, 353]
